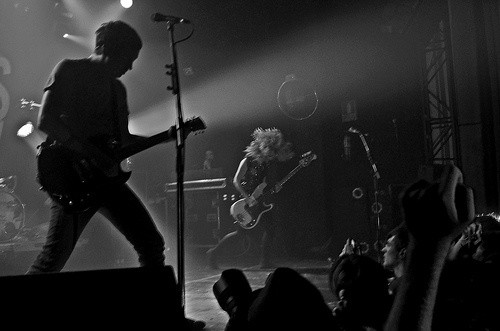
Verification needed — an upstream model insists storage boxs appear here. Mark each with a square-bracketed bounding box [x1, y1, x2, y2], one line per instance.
[164, 177, 227, 248]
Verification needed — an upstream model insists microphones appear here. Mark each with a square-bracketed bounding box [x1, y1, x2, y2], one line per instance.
[349, 127, 361, 134]
[150, 13, 190, 23]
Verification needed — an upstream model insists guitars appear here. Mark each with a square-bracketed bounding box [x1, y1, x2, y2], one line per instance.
[230, 150, 319, 231]
[38, 117, 207, 215]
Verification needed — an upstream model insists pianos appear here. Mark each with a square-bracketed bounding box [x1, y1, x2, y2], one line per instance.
[163, 177, 234, 253]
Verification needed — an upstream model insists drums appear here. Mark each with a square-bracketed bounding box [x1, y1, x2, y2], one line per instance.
[0, 188, 27, 243]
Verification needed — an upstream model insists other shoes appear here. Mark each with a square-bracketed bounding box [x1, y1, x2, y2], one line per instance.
[184, 315, 206, 331]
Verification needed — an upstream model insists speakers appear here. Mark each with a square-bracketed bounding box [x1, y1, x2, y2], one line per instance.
[0, 267, 177, 331]
[164, 189, 246, 259]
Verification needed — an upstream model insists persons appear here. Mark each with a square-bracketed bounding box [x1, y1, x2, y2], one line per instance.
[385, 166, 475, 331]
[202, 148, 219, 171]
[24, 20, 207, 331]
[233, 125, 296, 270]
[224, 212, 500, 331]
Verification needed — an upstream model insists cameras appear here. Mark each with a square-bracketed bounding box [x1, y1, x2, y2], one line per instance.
[213, 269, 253, 317]
